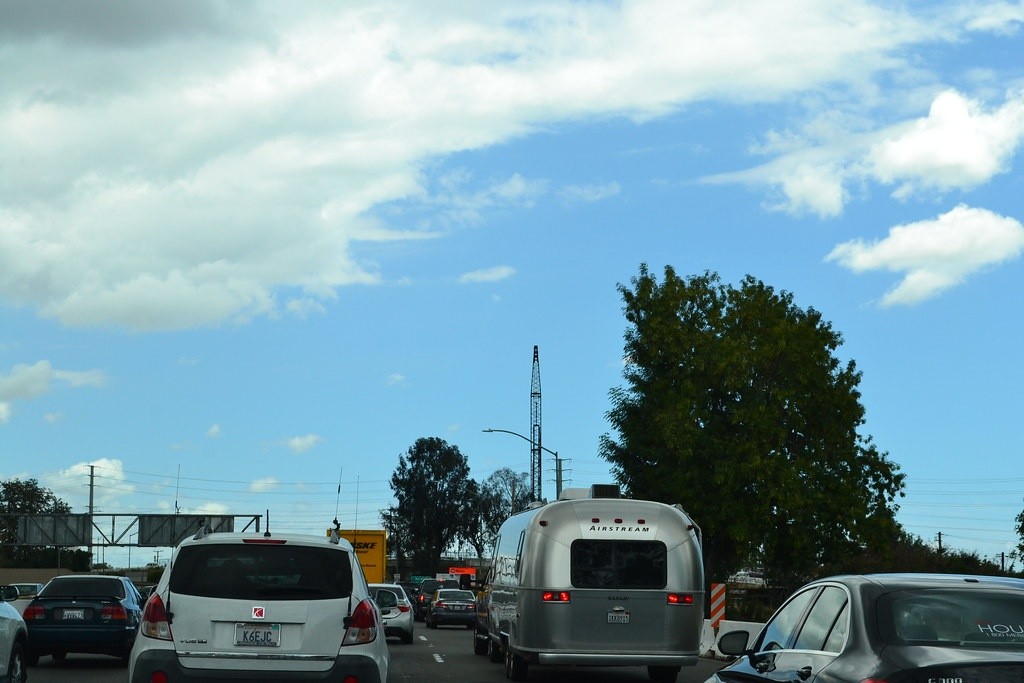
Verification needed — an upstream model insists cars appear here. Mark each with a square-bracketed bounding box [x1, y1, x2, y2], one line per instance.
[140, 585, 153, 599]
[425, 589, 477, 629]
[8, 583, 45, 600]
[703, 573, 1023, 683]
[0, 584, 32, 683]
[393, 580, 421, 600]
[22, 574, 146, 667]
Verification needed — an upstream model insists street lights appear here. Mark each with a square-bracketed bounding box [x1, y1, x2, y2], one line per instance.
[481, 427, 559, 501]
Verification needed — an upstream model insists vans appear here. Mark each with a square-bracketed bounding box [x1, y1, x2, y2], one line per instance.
[459, 497, 704, 683]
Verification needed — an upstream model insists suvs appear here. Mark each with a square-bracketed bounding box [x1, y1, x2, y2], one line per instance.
[127, 523, 391, 683]
[369, 582, 417, 643]
[414, 578, 461, 620]
